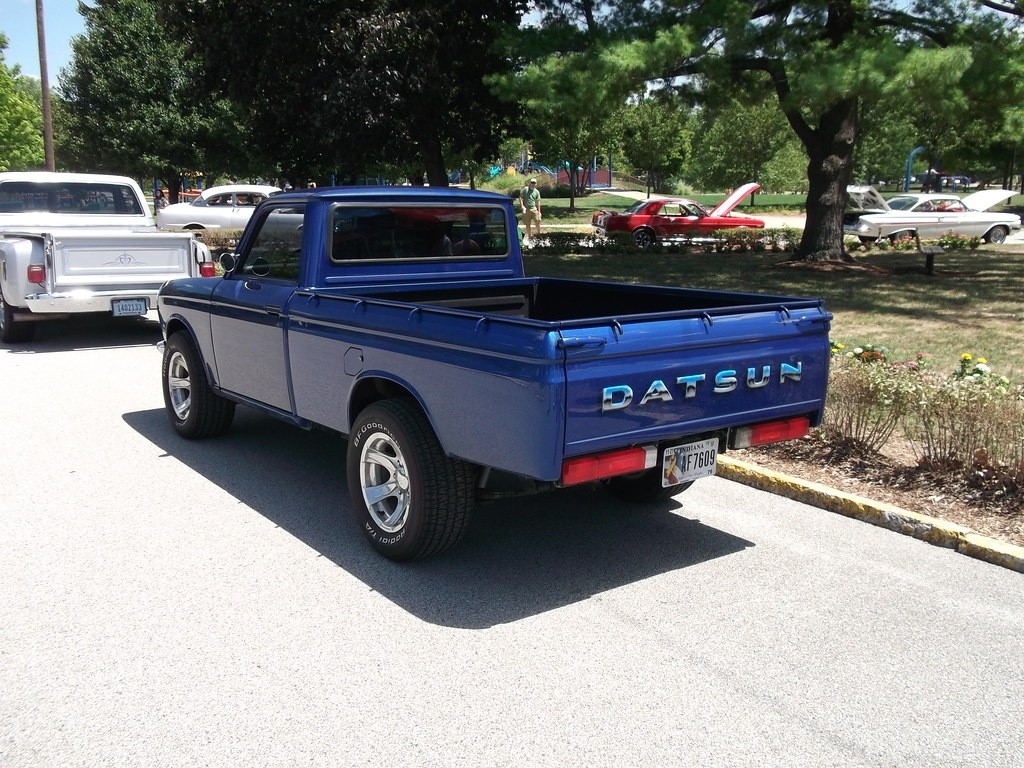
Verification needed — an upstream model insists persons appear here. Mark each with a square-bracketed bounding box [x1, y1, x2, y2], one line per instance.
[519, 178, 541, 239]
[153, 189, 170, 227]
[270, 180, 275, 187]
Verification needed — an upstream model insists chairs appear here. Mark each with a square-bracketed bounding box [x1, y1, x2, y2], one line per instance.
[358, 209, 446, 258]
[0, 201, 23, 213]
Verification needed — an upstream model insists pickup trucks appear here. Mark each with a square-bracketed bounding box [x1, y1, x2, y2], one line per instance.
[158, 186, 832, 564]
[0, 172, 215, 344]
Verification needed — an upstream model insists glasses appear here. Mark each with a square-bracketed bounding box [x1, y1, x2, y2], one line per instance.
[531, 182, 535, 184]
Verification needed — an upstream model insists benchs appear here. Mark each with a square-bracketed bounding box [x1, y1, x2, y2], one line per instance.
[915, 227, 945, 276]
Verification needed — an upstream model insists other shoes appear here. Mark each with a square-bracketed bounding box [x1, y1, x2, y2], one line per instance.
[528, 235, 542, 241]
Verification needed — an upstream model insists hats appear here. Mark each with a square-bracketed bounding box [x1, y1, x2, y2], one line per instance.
[530, 178, 537, 183]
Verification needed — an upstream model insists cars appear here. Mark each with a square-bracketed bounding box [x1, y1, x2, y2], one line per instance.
[592, 182, 765, 252]
[153, 184, 305, 236]
[842, 180, 1021, 248]
